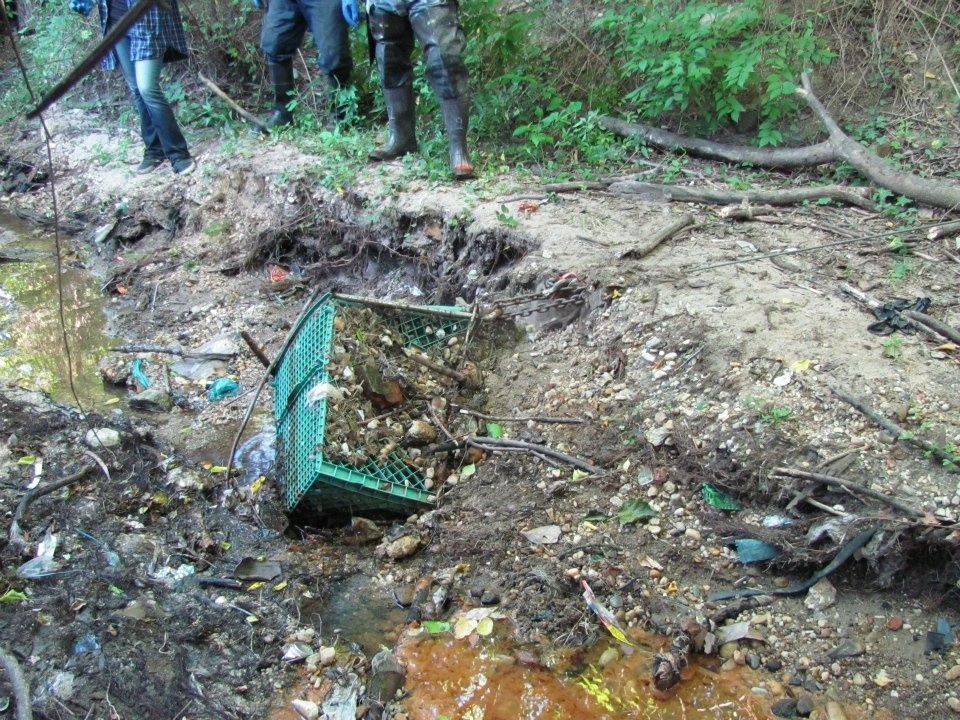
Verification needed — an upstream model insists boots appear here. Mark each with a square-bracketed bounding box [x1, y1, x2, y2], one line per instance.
[252, 82, 294, 133]
[442, 97, 475, 175]
[368, 88, 419, 160]
[324, 74, 349, 129]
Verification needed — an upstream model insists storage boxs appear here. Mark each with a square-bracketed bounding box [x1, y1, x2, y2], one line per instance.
[271, 294, 471, 517]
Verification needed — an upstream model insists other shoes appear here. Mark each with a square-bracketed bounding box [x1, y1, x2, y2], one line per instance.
[136, 159, 160, 174]
[171, 157, 195, 176]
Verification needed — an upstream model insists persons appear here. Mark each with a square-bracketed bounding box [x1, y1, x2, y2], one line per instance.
[251, 0, 358, 138]
[340, 0, 474, 176]
[68, 0, 194, 177]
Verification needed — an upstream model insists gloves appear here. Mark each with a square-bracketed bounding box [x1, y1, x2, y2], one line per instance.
[252, 0, 262, 9]
[341, 0, 358, 28]
